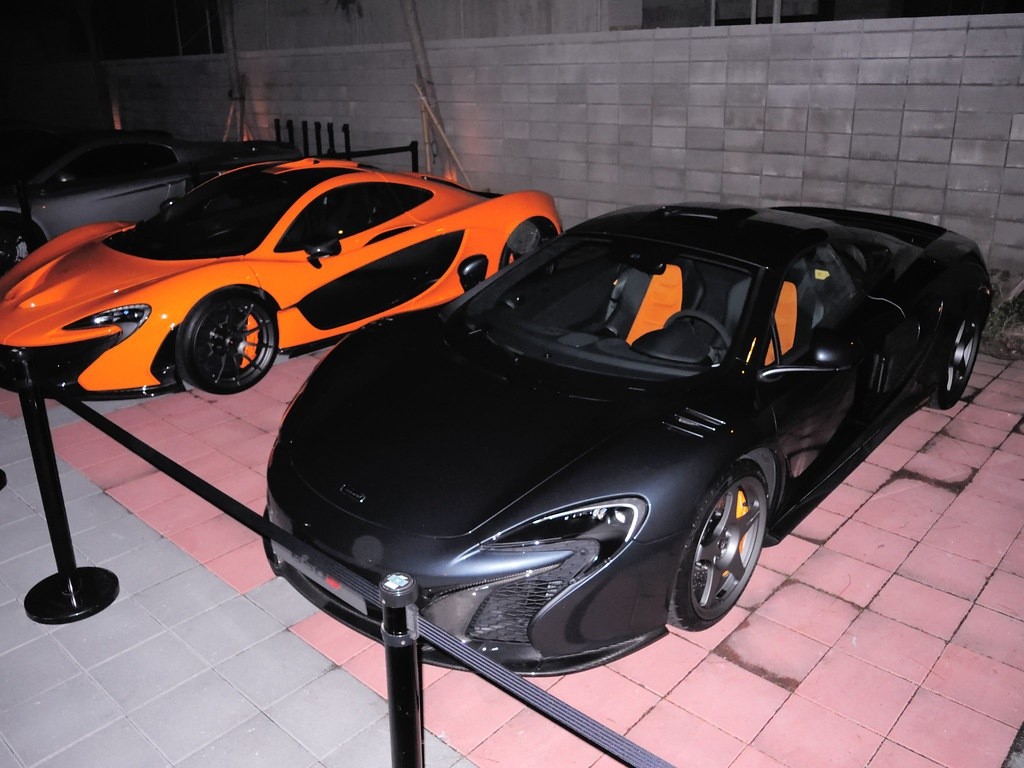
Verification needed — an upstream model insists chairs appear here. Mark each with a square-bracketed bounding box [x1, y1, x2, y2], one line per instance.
[295, 190, 351, 252]
[582, 251, 816, 375]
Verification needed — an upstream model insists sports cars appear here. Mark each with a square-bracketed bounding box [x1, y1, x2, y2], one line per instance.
[0, 158, 563, 399]
[265, 198, 994, 678]
[0, 130, 303, 279]
